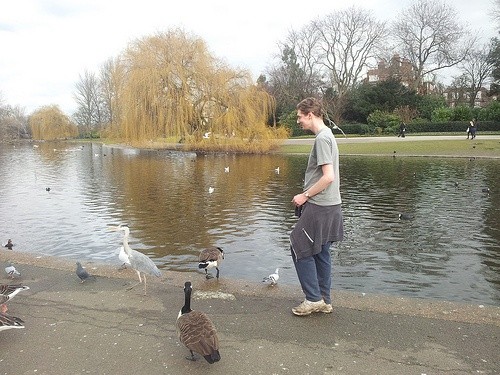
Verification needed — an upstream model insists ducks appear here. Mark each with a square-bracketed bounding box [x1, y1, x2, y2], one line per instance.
[45, 187, 51, 191]
[5, 238, 14, 248]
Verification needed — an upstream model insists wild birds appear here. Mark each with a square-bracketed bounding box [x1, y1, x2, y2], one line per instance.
[224, 166, 230, 174]
[5, 265, 22, 280]
[260, 267, 282, 286]
[76, 262, 95, 284]
[0, 281, 31, 332]
[111, 225, 162, 298]
[199, 246, 225, 281]
[176, 278, 221, 364]
[208, 186, 215, 194]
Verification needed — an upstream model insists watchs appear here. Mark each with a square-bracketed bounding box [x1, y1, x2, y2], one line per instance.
[304, 191, 311, 198]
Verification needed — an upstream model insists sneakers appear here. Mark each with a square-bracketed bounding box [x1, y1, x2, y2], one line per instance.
[291, 298, 326, 315]
[320, 304, 334, 313]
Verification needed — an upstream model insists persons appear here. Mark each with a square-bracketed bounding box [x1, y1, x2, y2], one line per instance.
[398, 121, 405, 137]
[466, 119, 476, 139]
[289, 97, 344, 316]
